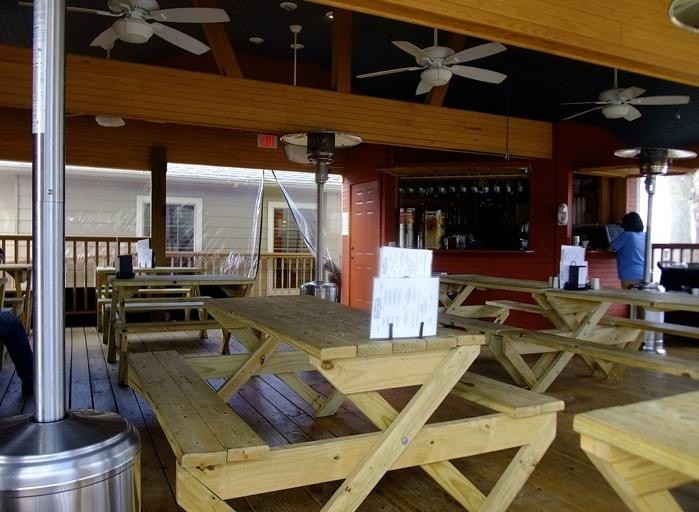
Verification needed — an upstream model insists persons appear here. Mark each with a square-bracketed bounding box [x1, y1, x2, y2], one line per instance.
[1, 310, 33, 398]
[607, 211, 647, 351]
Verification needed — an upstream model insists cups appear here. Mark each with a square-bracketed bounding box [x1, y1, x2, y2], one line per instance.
[549, 277, 558, 289]
[591, 278, 600, 290]
[582, 241, 589, 249]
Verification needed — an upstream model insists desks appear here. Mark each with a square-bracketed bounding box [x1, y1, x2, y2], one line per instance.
[200, 291, 482, 398]
[571, 388, 698, 512]
[435, 270, 699, 315]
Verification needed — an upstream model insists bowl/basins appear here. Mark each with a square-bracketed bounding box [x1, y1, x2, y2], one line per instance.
[389, 241, 397, 247]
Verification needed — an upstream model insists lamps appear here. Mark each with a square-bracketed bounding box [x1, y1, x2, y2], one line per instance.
[602, 103, 630, 119]
[112, 18, 154, 44]
[420, 68, 452, 87]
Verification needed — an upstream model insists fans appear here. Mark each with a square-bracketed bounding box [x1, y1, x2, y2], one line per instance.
[67, 0, 231, 56]
[356, 27, 507, 96]
[558, 69, 690, 122]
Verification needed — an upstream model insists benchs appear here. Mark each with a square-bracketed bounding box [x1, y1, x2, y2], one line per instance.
[437, 296, 699, 384]
[123, 347, 275, 475]
[1, 265, 261, 390]
[447, 368, 567, 425]
